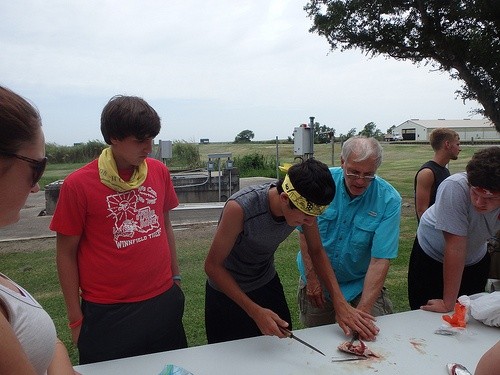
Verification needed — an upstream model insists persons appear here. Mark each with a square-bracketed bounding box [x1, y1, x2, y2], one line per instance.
[204, 155, 378, 344]
[414, 129, 461, 228]
[296, 135, 402, 338]
[407, 146, 500, 312]
[50, 95, 188, 364]
[0, 88, 80, 374]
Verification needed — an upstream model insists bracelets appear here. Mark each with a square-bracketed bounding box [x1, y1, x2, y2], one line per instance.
[172, 275, 182, 281]
[68, 315, 84, 328]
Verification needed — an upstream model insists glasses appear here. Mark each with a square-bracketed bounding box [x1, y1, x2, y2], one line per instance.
[4, 152, 47, 185]
[344, 167, 375, 181]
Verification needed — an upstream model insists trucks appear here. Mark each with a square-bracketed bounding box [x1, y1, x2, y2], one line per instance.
[384, 132, 403, 141]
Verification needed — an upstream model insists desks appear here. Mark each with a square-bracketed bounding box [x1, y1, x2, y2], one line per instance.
[72, 309, 500, 375]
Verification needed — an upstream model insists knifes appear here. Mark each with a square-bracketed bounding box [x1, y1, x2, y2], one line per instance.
[277, 324, 326, 356]
[348, 332, 358, 350]
